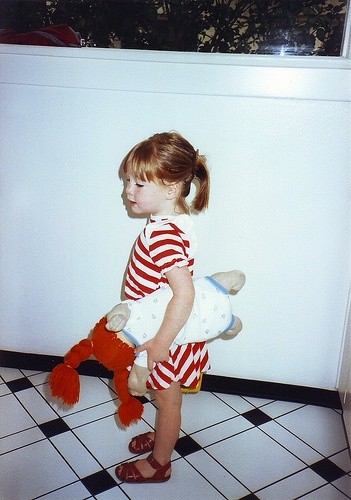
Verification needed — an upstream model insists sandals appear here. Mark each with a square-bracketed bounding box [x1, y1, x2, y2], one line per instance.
[128, 431, 155, 454]
[115, 452, 171, 483]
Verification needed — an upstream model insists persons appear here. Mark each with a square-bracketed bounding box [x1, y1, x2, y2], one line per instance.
[115, 130, 211, 484]
